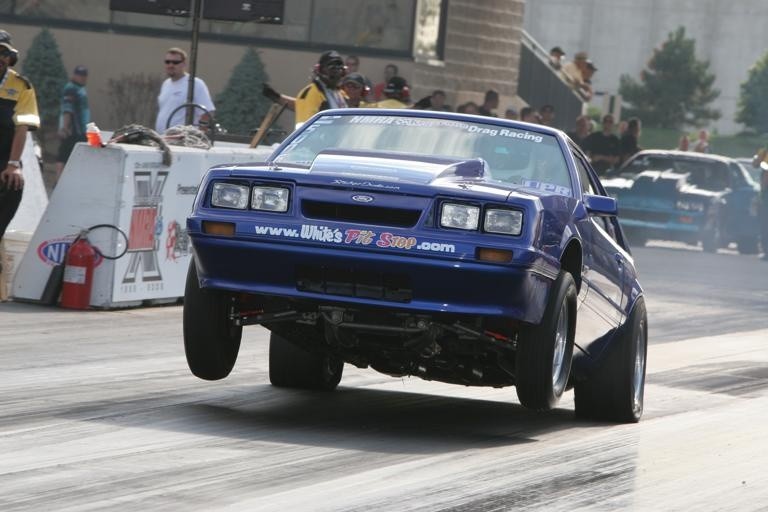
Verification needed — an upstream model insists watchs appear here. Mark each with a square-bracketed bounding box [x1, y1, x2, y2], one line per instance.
[7, 160, 21, 168]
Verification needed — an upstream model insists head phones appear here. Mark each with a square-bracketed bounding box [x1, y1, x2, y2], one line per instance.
[384, 79, 411, 96]
[315, 56, 349, 75]
[338, 80, 371, 96]
[9, 51, 18, 68]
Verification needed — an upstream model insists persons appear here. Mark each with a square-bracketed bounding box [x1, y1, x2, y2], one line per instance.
[695, 128, 711, 153]
[294, 49, 556, 138]
[549, 47, 646, 178]
[0, 29, 41, 272]
[751, 142, 767, 262]
[154, 48, 216, 146]
[676, 134, 691, 152]
[57, 63, 90, 164]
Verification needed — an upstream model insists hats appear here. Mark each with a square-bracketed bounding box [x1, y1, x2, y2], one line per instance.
[0, 29, 20, 66]
[75, 65, 88, 76]
[319, 50, 342, 71]
[551, 47, 598, 71]
[382, 77, 406, 93]
[343, 73, 364, 87]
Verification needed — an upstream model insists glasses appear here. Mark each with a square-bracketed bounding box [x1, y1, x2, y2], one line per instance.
[327, 64, 349, 79]
[164, 60, 182, 65]
[1, 50, 11, 56]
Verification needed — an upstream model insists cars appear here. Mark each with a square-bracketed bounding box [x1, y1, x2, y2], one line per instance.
[178, 104, 647, 422]
[588, 148, 765, 260]
[734, 151, 765, 191]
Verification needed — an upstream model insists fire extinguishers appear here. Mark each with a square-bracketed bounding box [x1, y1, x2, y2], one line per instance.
[59, 223, 129, 310]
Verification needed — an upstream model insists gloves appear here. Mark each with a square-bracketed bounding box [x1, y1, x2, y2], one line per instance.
[262, 82, 279, 104]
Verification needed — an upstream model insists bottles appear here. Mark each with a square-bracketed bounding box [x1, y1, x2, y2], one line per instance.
[85, 121, 102, 146]
